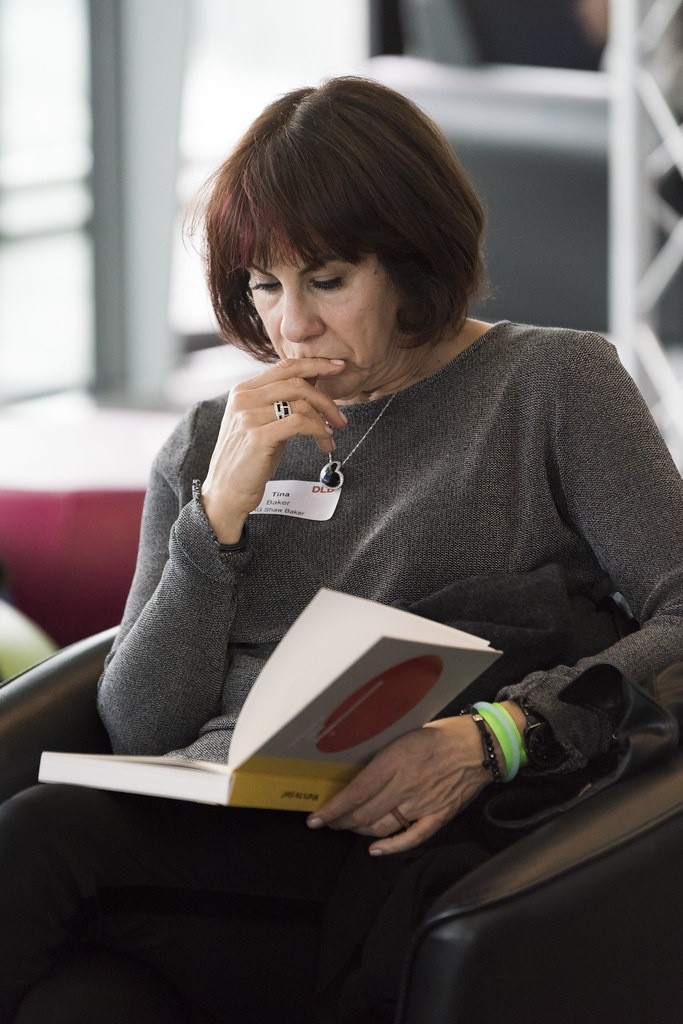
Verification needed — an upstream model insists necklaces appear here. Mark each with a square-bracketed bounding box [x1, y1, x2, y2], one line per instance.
[320, 343, 440, 489]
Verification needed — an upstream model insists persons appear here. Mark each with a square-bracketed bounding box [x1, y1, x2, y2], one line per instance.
[1, 77, 683, 1024]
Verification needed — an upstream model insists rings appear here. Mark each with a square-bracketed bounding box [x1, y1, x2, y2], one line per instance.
[274, 401, 291, 420]
[392, 807, 410, 828]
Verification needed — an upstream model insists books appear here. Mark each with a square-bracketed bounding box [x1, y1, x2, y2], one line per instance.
[39, 587, 504, 812]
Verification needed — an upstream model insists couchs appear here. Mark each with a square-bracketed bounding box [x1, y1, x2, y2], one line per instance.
[0, 626, 682, 1024]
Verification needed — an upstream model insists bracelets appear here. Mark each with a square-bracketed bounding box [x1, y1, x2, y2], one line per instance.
[460, 704, 500, 784]
[218, 522, 253, 553]
[478, 702, 528, 775]
[472, 701, 520, 783]
